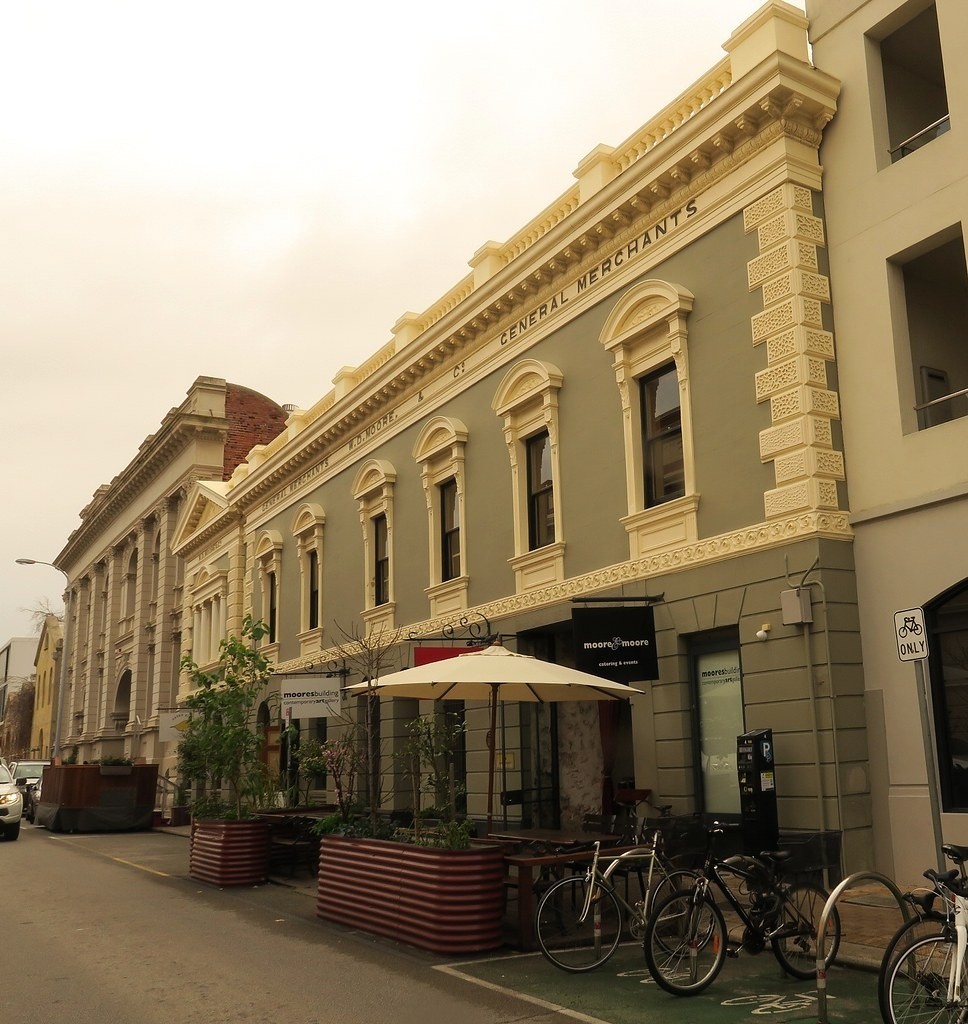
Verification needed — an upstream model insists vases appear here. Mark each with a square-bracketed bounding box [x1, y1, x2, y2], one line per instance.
[100, 765, 133, 776]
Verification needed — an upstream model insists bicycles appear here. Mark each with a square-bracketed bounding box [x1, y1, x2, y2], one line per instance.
[534, 828, 717, 974]
[643, 819, 847, 995]
[608, 784, 679, 847]
[877, 844, 968, 1024]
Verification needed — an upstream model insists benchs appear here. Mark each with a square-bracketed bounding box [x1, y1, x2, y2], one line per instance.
[272, 835, 311, 880]
[504, 861, 555, 938]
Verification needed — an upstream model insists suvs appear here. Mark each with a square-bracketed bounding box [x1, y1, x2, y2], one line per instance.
[8, 760, 53, 824]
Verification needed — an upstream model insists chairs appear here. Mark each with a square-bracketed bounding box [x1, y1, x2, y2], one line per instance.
[540, 813, 680, 922]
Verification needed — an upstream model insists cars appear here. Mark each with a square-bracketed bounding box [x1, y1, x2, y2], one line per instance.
[0, 759, 27, 841]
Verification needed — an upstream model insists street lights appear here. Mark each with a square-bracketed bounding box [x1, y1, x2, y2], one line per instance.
[15, 558, 72, 759]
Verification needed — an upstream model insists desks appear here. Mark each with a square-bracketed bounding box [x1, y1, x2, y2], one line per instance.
[280, 812, 364, 873]
[487, 827, 622, 930]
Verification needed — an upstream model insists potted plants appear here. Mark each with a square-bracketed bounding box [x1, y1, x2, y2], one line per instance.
[152, 614, 508, 957]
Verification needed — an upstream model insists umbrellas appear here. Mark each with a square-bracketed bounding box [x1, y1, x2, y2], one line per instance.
[340, 642, 647, 838]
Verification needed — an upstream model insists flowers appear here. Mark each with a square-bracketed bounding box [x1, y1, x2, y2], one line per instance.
[319, 738, 363, 824]
[99, 750, 138, 766]
